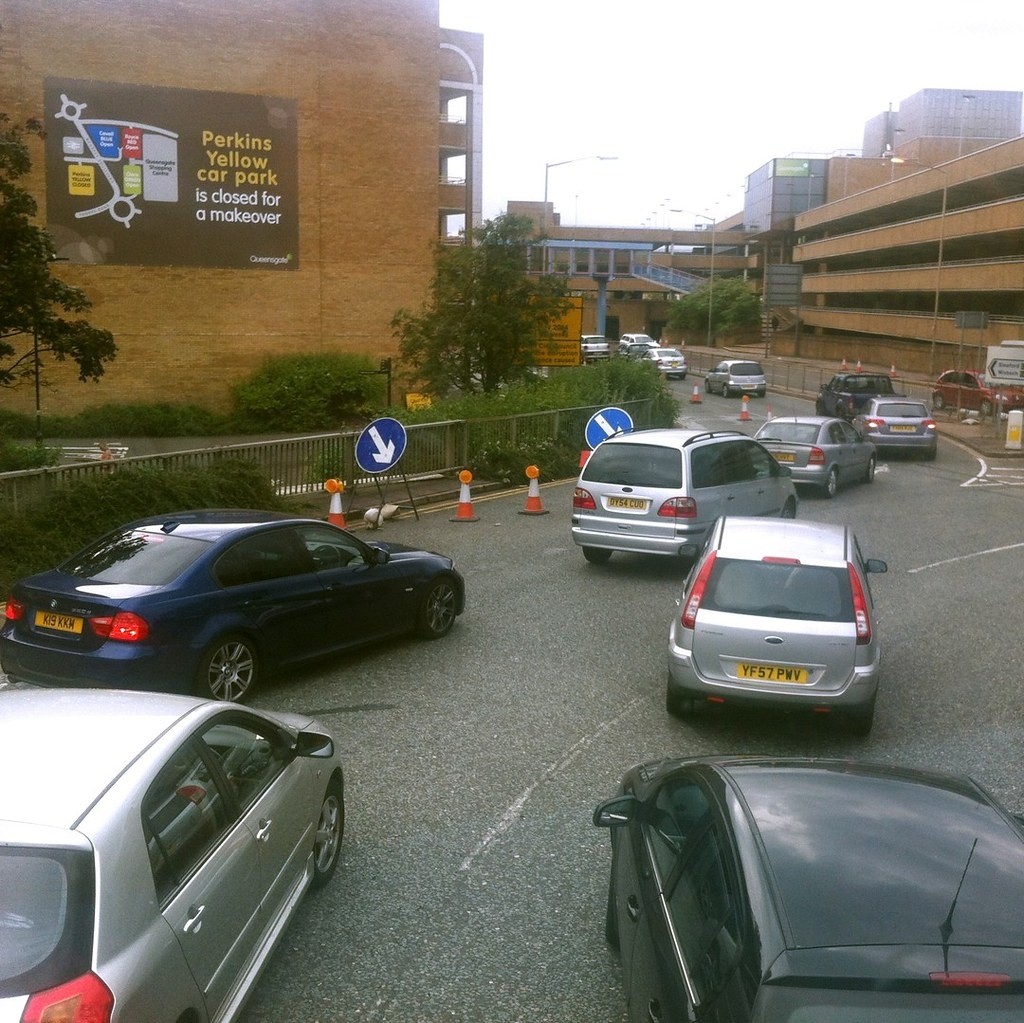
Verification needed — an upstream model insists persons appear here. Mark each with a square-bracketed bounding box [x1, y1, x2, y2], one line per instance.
[98, 440, 115, 476]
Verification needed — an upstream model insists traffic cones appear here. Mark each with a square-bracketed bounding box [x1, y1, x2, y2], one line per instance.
[854, 357, 863, 374]
[324, 478, 358, 533]
[517, 465, 551, 516]
[449, 470, 481, 523]
[736, 395, 753, 422]
[890, 362, 900, 379]
[838, 355, 848, 372]
[689, 379, 705, 404]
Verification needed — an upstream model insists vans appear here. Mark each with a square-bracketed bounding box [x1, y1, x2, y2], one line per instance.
[572, 424, 799, 565]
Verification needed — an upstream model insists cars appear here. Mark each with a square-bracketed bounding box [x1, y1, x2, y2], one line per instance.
[0, 509, 464, 706]
[617, 334, 688, 381]
[932, 368, 1024, 418]
[666, 512, 888, 735]
[704, 359, 767, 398]
[593, 753, 1024, 1023]
[852, 396, 939, 461]
[752, 416, 878, 499]
[0, 688, 344, 1022]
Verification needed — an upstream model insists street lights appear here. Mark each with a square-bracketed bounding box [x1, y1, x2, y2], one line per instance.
[749, 224, 769, 356]
[670, 208, 716, 348]
[890, 157, 948, 374]
[541, 154, 619, 275]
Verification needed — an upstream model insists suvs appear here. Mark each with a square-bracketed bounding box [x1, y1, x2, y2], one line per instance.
[580, 335, 611, 366]
[815, 370, 895, 423]
